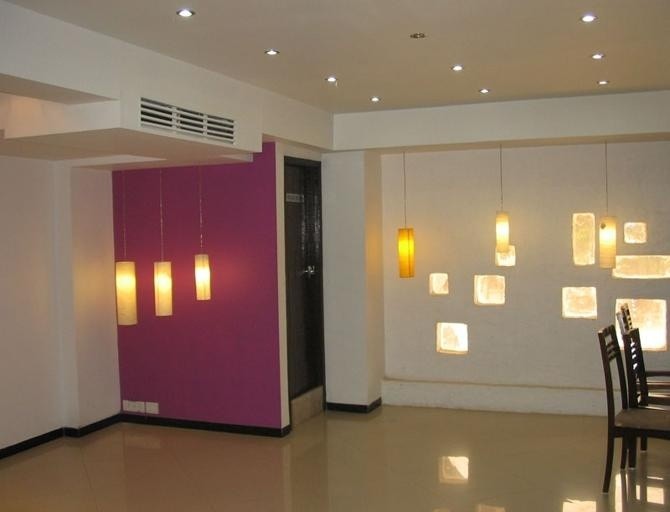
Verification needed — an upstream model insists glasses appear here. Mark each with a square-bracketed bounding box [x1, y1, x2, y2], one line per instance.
[153, 168, 173, 316]
[194, 165, 210, 301]
[495, 143, 510, 253]
[397, 151, 416, 279]
[599, 140, 617, 270]
[116, 169, 137, 326]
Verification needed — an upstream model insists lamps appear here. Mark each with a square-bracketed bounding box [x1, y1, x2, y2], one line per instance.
[598, 303, 670, 492]
